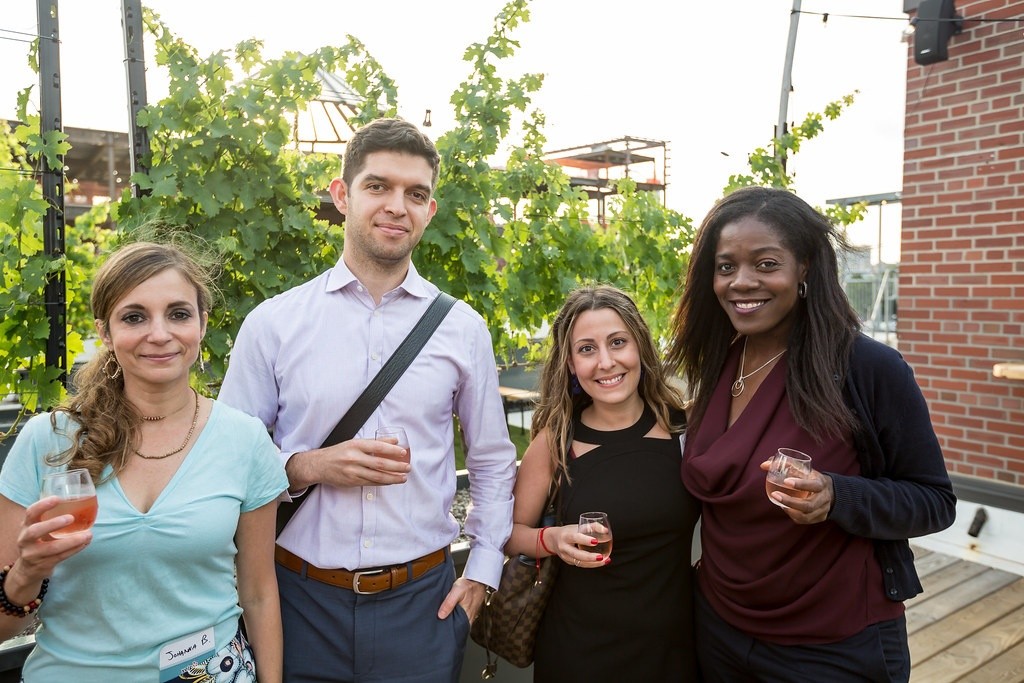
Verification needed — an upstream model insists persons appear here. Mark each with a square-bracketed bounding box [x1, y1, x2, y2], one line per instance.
[216, 119, 517, 683]
[660, 187, 958, 682]
[505, 286, 708, 683]
[0, 241, 290, 683]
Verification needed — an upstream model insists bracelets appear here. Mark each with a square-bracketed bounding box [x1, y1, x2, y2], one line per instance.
[484, 584, 493, 606]
[535, 528, 542, 587]
[0, 563, 49, 619]
[540, 526, 555, 554]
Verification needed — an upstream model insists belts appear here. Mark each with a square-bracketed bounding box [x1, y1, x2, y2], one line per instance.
[273, 543, 452, 594]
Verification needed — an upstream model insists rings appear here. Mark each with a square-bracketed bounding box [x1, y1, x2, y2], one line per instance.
[573, 558, 580, 567]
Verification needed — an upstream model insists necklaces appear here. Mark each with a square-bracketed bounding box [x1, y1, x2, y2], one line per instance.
[730, 336, 789, 397]
[141, 395, 194, 420]
[132, 386, 199, 458]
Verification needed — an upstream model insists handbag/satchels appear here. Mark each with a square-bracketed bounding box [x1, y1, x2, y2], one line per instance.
[468, 417, 575, 668]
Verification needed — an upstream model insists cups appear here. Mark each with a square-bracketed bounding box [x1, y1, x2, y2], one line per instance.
[40, 468, 98, 538]
[374, 426, 410, 474]
[576, 512, 613, 560]
[765, 448, 813, 506]
